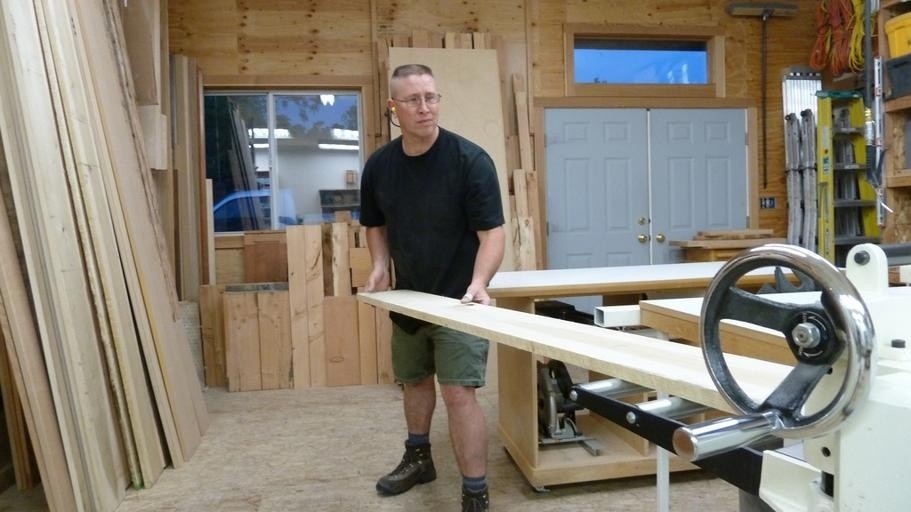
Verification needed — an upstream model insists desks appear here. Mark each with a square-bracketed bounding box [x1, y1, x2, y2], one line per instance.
[486, 237, 848, 494]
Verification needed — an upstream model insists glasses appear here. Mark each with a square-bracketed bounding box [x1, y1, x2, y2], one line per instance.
[392, 93, 442, 106]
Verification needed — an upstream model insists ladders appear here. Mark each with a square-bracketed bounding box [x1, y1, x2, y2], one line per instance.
[784, 91, 880, 268]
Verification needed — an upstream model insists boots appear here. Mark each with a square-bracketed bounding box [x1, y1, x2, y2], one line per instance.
[376, 440, 436, 495]
[461, 485, 489, 511]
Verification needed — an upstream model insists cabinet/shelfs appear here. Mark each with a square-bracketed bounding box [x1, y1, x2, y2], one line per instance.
[877, 1, 911, 247]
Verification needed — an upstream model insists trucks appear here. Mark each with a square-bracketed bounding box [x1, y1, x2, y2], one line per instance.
[212, 189, 300, 233]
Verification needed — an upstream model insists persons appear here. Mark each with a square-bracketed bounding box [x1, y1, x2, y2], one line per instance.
[356, 63, 508, 512]
